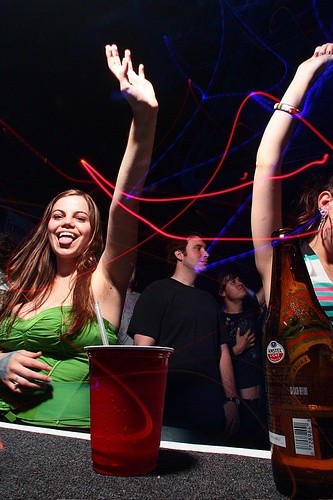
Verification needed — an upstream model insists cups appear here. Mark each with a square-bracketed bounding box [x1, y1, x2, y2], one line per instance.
[83, 344, 174, 478]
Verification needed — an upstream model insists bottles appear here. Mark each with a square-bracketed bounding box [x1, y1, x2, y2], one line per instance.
[264, 228, 333, 500]
